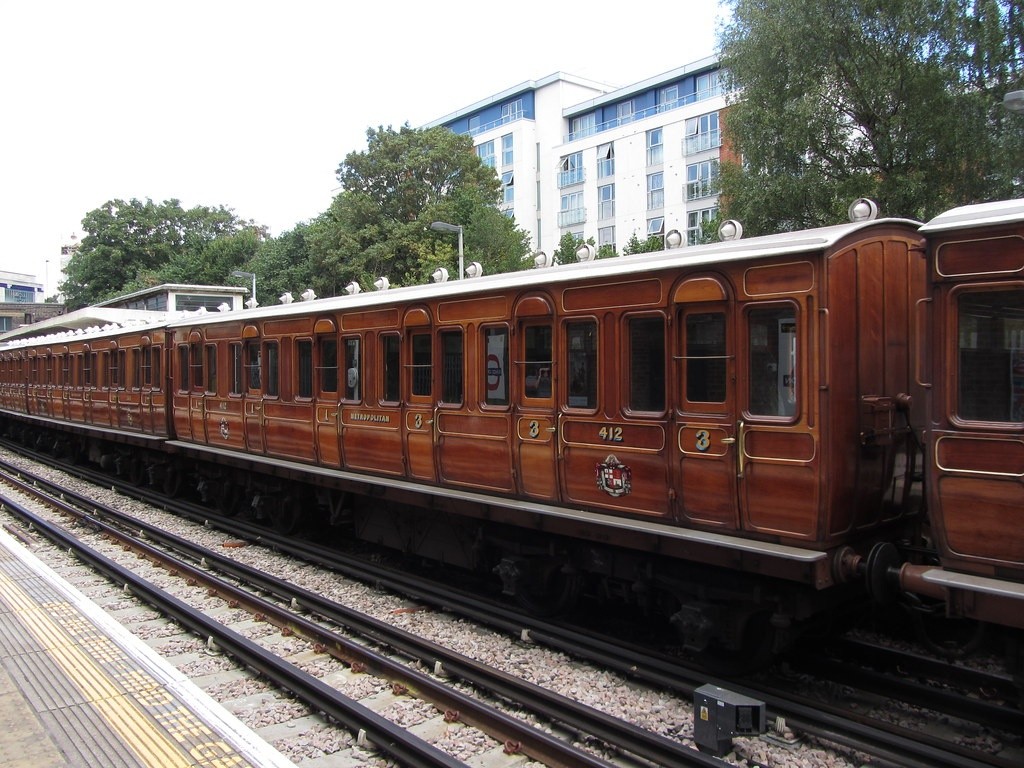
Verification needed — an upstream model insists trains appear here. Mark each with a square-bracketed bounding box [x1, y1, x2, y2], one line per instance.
[0, 195, 1023, 681]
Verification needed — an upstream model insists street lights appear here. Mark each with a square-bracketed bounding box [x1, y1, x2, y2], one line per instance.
[46, 260, 50, 299]
[231, 271, 256, 309]
[430, 222, 465, 281]
